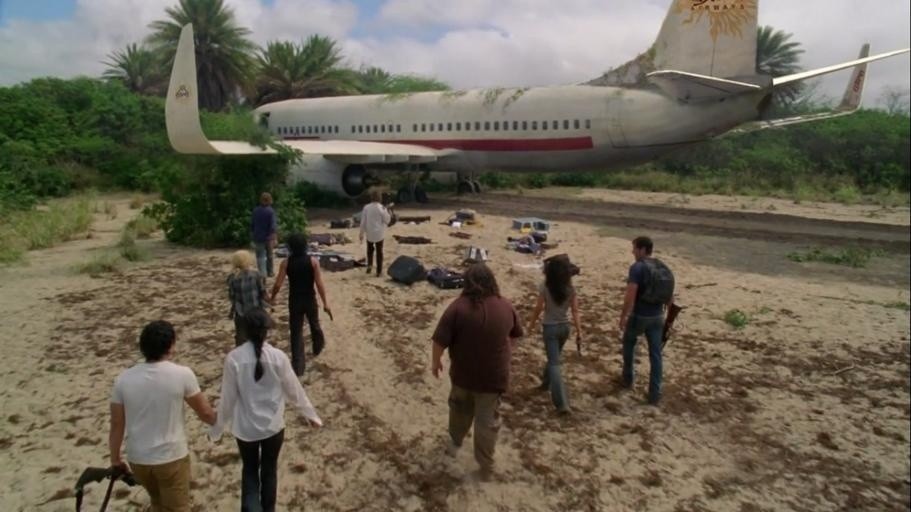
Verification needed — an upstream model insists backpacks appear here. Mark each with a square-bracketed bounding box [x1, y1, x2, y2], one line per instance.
[638, 256, 675, 306]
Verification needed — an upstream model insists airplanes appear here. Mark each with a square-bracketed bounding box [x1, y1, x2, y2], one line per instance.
[166, 1, 910, 205]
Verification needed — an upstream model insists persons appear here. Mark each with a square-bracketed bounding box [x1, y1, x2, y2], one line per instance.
[620, 236, 674, 403]
[268, 234, 333, 376]
[526, 253, 581, 412]
[110, 318, 217, 511]
[431, 262, 523, 482]
[208, 307, 323, 511]
[359, 186, 397, 277]
[250, 192, 277, 277]
[227, 250, 275, 346]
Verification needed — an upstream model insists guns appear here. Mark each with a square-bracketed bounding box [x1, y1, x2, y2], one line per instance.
[73, 468, 139, 488]
[664, 303, 681, 344]
[576, 338, 582, 358]
[324, 307, 334, 321]
